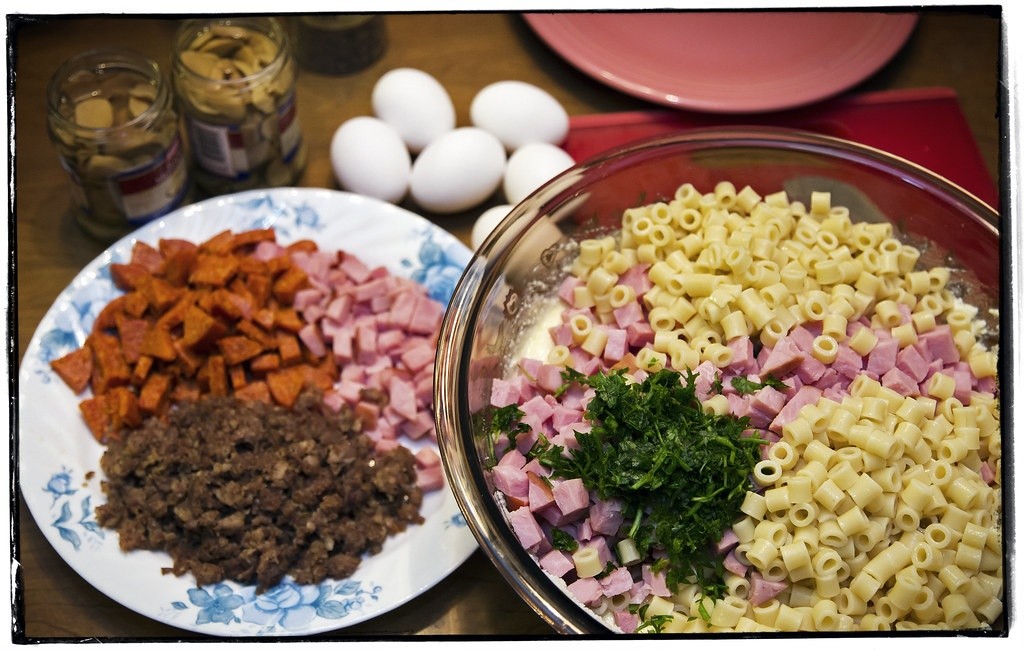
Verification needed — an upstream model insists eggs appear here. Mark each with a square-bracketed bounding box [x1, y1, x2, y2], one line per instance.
[330, 70, 588, 277]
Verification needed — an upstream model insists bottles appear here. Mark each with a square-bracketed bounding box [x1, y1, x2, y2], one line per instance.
[296, 14, 388, 78]
[170, 17, 307, 197]
[45, 48, 191, 241]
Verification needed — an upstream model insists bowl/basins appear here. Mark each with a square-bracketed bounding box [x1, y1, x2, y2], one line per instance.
[433, 127, 1003, 636]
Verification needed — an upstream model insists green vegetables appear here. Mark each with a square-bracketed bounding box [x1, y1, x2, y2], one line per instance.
[481, 357, 793, 635]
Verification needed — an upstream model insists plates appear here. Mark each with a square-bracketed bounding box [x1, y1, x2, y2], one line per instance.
[16, 187, 500, 637]
[520, 12, 917, 115]
